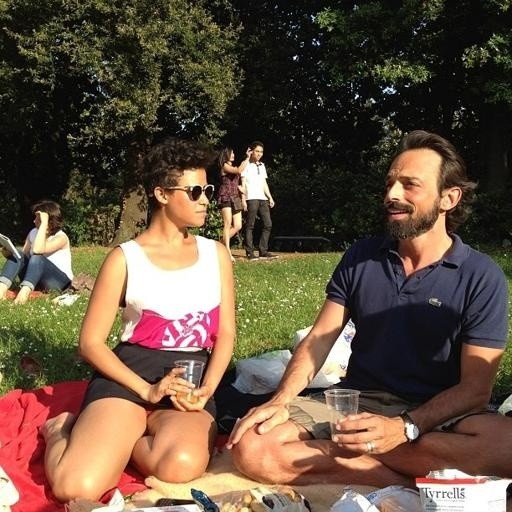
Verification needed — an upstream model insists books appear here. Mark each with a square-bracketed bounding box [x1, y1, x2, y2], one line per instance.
[0, 233, 22, 260]
[51, 293, 79, 307]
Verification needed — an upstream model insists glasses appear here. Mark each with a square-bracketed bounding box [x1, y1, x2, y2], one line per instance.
[162, 183, 215, 202]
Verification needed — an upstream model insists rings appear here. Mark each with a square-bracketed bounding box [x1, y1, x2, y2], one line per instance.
[366, 441, 374, 453]
[168, 383, 174, 389]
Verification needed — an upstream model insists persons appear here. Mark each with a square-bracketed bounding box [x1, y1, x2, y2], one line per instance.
[240, 142, 279, 261]
[226, 129, 512, 491]
[216, 147, 254, 264]
[38, 139, 235, 503]
[0, 200, 73, 307]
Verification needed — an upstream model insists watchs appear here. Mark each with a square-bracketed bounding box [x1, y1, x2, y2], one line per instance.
[398, 410, 420, 443]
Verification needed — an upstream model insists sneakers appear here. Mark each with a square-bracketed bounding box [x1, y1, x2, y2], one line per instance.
[247, 256, 258, 261]
[258, 254, 279, 260]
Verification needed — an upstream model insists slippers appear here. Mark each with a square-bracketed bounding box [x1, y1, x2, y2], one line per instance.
[20, 354, 42, 379]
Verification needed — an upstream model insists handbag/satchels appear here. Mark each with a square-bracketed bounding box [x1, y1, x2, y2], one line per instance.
[72, 270, 95, 292]
[233, 197, 245, 210]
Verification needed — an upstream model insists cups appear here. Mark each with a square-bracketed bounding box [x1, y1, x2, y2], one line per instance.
[173, 359, 206, 405]
[324, 388, 360, 443]
